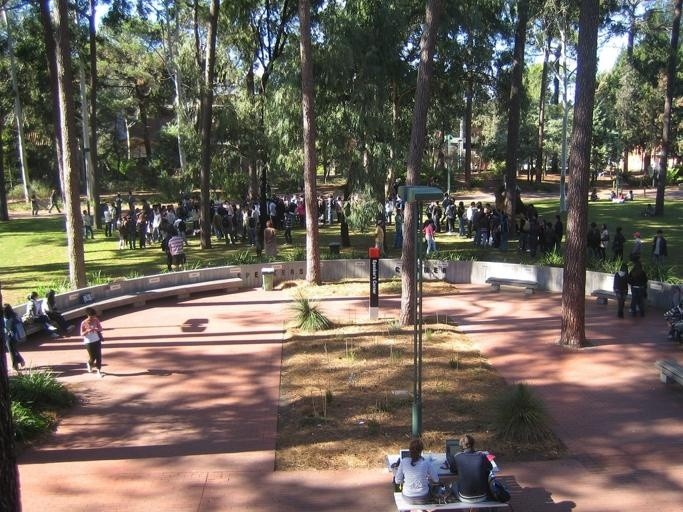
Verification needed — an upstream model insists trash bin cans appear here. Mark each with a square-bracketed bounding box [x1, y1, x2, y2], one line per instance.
[261, 268, 275, 291]
[328, 242, 340, 255]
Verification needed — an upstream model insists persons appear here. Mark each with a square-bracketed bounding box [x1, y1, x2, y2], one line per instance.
[421, 190, 567, 266]
[590, 185, 656, 215]
[449, 433, 493, 506]
[29, 192, 40, 217]
[627, 262, 648, 316]
[395, 441, 440, 506]
[588, 221, 675, 269]
[663, 302, 682, 339]
[47, 188, 62, 215]
[316, 192, 405, 255]
[80, 309, 106, 378]
[2, 290, 70, 372]
[207, 192, 305, 260]
[613, 264, 629, 320]
[81, 188, 200, 272]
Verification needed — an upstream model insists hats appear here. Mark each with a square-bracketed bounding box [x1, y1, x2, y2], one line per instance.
[631, 231, 641, 237]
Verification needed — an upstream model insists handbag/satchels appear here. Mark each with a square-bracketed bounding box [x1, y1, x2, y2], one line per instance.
[487, 476, 511, 503]
[97, 332, 104, 342]
[429, 486, 457, 504]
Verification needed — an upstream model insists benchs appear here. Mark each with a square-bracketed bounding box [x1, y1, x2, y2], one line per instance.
[485, 276, 541, 295]
[651, 358, 682, 389]
[591, 289, 632, 306]
[10, 264, 243, 337]
[393, 491, 509, 512]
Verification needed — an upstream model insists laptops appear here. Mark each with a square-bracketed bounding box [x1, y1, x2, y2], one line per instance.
[448, 446, 462, 458]
[400, 449, 410, 460]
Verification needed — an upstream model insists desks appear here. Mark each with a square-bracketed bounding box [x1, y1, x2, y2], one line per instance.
[384, 451, 499, 478]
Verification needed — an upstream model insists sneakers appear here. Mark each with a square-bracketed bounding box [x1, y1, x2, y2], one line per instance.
[86, 361, 93, 372]
[46, 324, 58, 332]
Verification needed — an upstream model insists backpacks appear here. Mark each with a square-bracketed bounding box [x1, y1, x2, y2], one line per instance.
[178, 221, 187, 232]
[222, 217, 229, 228]
[522, 220, 531, 233]
[10, 319, 27, 344]
